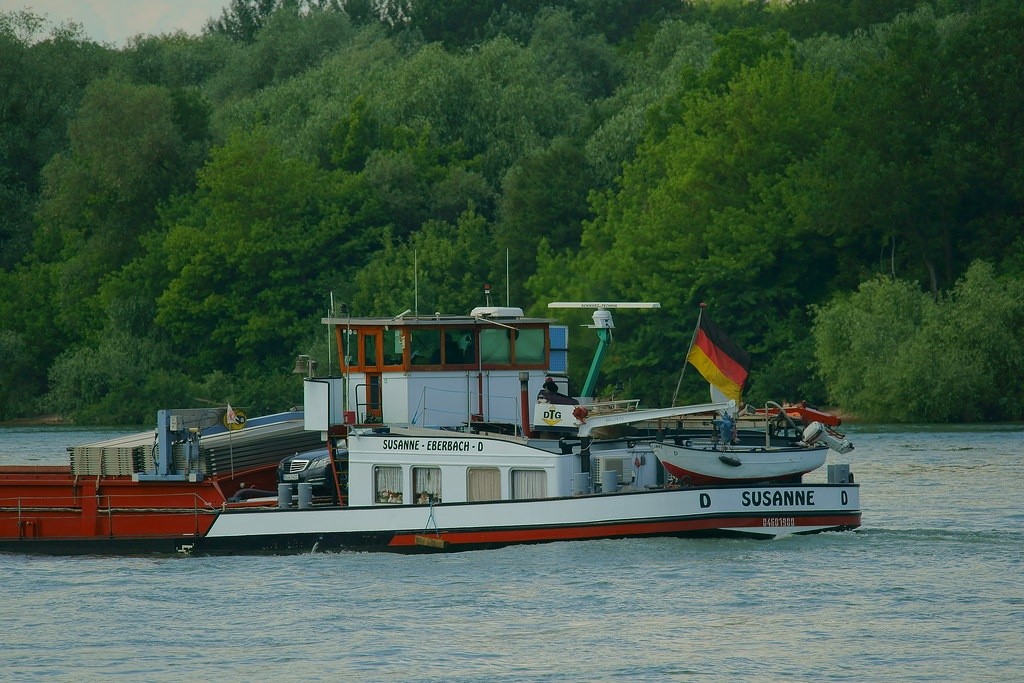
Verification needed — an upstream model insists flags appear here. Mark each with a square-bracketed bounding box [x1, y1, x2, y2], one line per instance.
[227, 404, 239, 424]
[686, 303, 755, 407]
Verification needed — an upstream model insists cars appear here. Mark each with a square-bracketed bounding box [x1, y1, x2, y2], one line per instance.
[278, 428, 391, 497]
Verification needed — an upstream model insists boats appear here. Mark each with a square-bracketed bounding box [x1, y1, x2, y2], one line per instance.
[572, 402, 777, 438]
[649, 422, 857, 484]
[192, 285, 862, 554]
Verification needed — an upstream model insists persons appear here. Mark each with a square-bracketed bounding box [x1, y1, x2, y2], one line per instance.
[428, 333, 458, 364]
[779, 399, 807, 409]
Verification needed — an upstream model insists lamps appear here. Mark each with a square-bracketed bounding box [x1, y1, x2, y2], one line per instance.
[293, 357, 307, 374]
